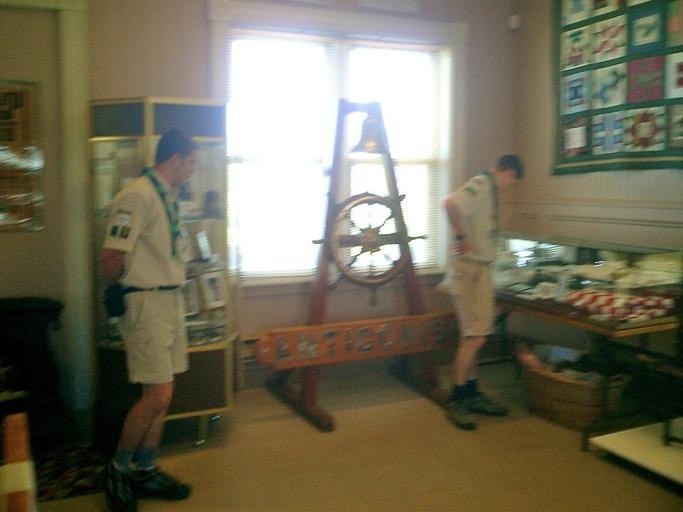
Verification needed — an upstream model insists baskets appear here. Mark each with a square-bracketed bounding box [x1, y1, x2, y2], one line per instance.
[522, 367, 633, 431]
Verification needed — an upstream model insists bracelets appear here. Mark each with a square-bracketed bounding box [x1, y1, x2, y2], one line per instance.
[453, 233, 468, 241]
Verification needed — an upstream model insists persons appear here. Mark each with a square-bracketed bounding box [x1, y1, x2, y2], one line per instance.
[99, 127, 193, 511]
[434, 154, 524, 430]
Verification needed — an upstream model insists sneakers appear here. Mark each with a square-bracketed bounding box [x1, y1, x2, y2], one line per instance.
[106, 464, 138, 512]
[134, 467, 189, 500]
[470, 391, 506, 416]
[448, 400, 476, 430]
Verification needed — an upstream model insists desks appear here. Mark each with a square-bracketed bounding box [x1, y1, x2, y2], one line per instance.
[495, 293, 682, 456]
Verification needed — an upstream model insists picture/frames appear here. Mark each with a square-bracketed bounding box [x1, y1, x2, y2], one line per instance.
[198, 271, 228, 311]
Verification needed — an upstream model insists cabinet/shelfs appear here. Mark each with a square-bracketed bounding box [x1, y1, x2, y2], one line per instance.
[85, 97, 239, 446]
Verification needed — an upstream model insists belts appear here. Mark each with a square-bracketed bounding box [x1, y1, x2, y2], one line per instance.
[122, 286, 178, 295]
